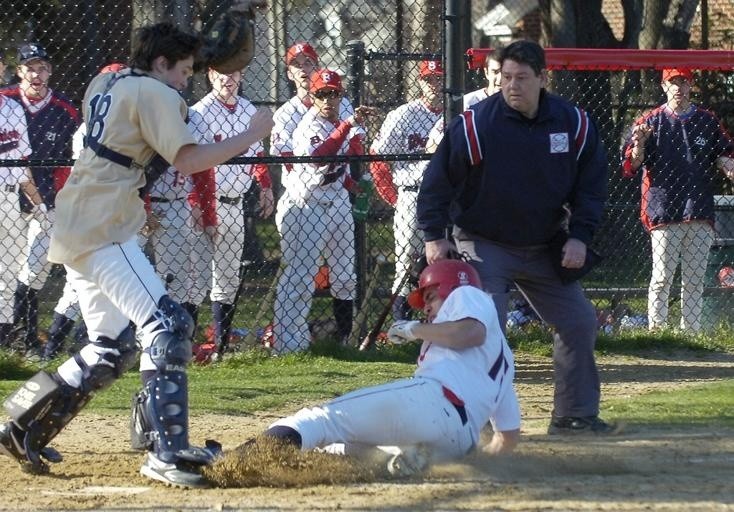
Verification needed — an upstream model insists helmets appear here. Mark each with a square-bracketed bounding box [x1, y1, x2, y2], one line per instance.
[406, 260, 480, 308]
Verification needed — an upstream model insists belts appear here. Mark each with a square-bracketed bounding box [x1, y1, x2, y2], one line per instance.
[401, 185, 418, 192]
[150, 196, 186, 202]
[3, 184, 15, 194]
[218, 196, 242, 205]
[442, 386, 468, 426]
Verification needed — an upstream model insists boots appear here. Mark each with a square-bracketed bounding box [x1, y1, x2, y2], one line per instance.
[375, 296, 412, 352]
[333, 298, 353, 348]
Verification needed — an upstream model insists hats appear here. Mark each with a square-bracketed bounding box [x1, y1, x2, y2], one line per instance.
[419, 60, 442, 77]
[18, 43, 50, 66]
[309, 71, 343, 93]
[286, 43, 316, 65]
[662, 68, 693, 83]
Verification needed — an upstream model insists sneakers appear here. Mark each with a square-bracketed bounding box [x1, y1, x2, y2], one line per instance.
[548, 416, 613, 435]
[0, 419, 51, 476]
[388, 440, 431, 478]
[141, 445, 217, 489]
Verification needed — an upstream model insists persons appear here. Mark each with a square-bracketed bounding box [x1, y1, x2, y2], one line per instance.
[1, 25, 274, 484]
[622, 69, 734, 331]
[237, 259, 520, 479]
[416, 42, 616, 432]
[143, 41, 444, 355]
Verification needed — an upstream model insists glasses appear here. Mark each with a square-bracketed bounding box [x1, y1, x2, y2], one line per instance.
[314, 89, 339, 100]
[421, 75, 443, 81]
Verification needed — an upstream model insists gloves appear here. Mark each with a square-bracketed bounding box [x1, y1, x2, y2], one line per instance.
[387, 320, 420, 344]
